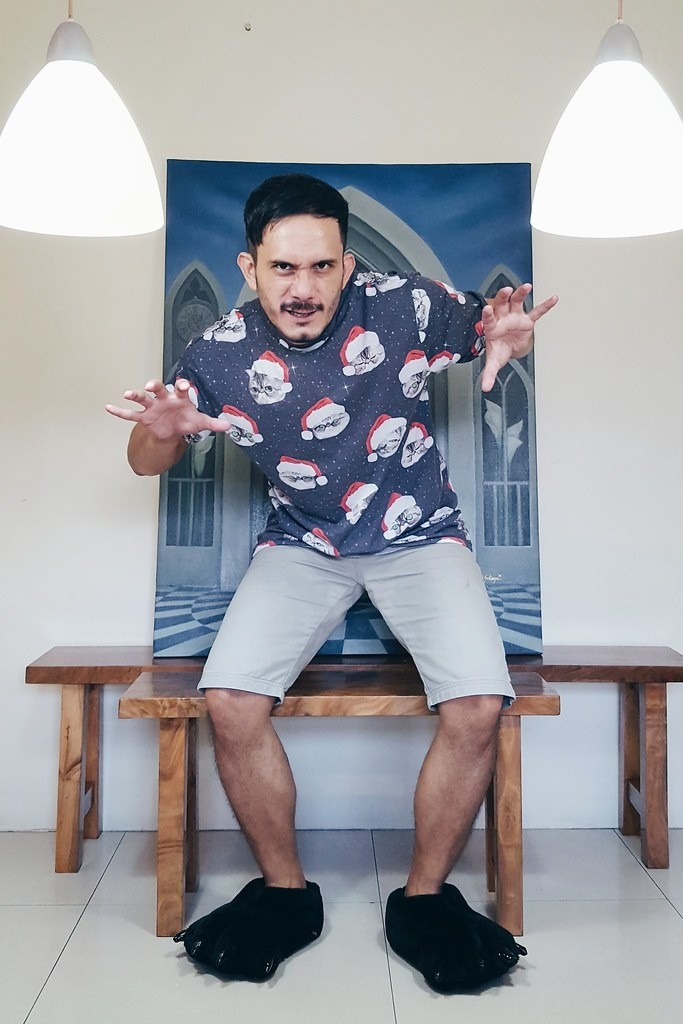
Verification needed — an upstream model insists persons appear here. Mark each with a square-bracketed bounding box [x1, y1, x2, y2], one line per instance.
[103, 170, 559, 997]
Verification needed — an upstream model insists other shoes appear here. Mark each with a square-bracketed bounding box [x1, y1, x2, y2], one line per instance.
[173, 873, 330, 982]
[385, 884, 530, 993]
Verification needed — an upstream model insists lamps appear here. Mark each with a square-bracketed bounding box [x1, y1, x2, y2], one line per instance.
[0, 1, 166, 238]
[526, 0, 683, 239]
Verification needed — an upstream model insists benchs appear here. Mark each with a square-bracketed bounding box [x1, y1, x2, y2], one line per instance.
[24, 645, 681, 887]
[117, 668, 561, 937]
